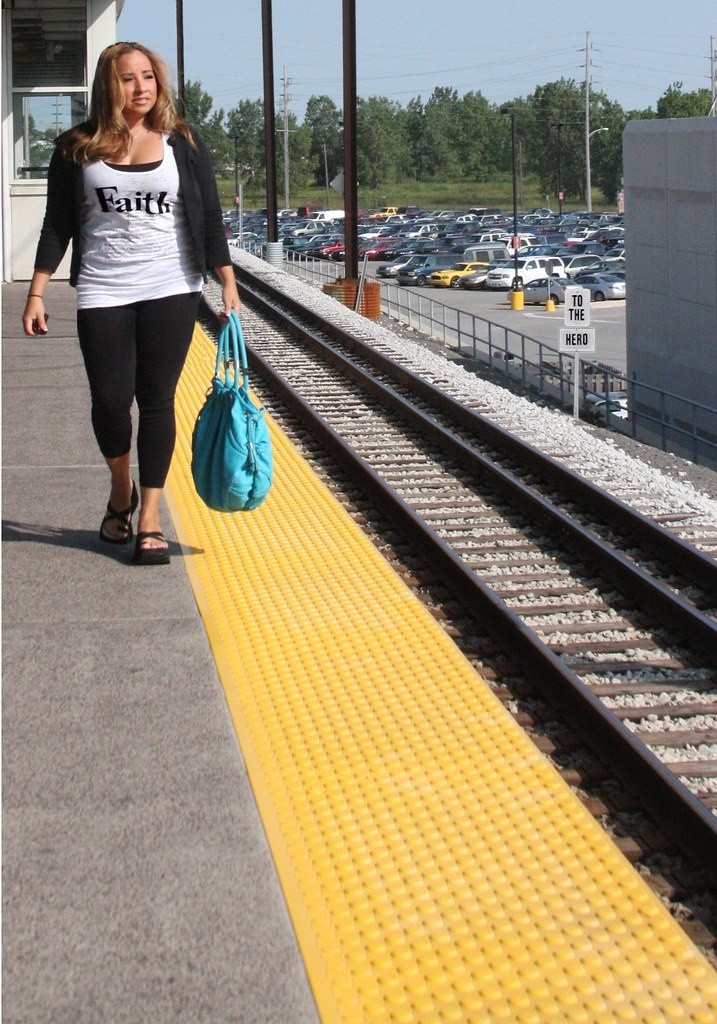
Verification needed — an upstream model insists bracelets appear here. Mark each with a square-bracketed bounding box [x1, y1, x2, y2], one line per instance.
[27, 294, 43, 298]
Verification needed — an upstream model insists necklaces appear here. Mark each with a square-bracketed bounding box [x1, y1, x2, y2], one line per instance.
[132, 125, 146, 140]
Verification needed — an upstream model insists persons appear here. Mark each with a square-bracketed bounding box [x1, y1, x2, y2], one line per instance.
[22, 42, 241, 565]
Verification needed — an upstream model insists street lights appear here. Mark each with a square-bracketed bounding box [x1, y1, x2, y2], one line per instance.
[586, 128, 609, 211]
[500, 108, 524, 310]
[229, 135, 243, 217]
[552, 121, 564, 218]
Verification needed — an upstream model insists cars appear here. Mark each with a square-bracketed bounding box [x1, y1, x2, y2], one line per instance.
[221, 205, 628, 287]
[574, 274, 626, 302]
[507, 277, 584, 304]
[585, 391, 629, 421]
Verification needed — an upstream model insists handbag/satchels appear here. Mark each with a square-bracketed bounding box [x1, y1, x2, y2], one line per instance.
[191, 309, 273, 512]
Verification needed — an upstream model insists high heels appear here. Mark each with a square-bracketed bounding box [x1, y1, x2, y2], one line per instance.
[99, 477, 138, 544]
[133, 529, 170, 565]
